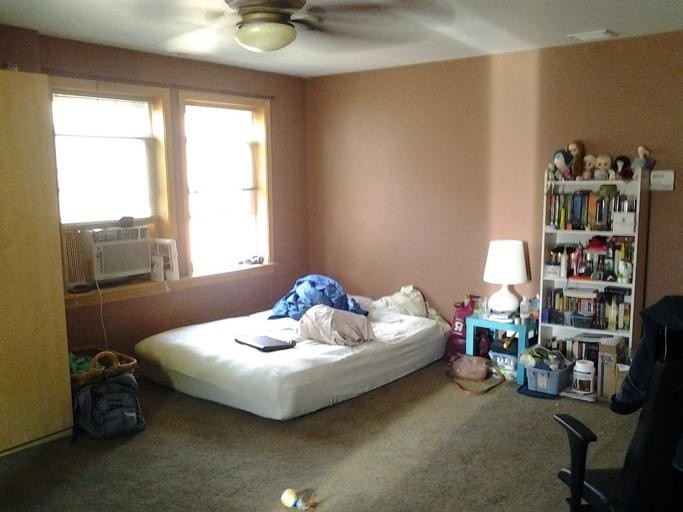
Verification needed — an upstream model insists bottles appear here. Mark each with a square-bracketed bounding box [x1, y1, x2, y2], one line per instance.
[573, 360, 595, 395]
[519, 295, 529, 321]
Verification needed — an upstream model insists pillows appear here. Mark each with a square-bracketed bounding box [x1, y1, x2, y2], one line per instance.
[370, 286, 429, 317]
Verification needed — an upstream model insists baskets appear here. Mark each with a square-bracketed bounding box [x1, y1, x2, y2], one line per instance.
[70, 346, 137, 430]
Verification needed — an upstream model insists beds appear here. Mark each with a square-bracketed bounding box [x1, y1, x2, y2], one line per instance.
[134, 294, 451, 422]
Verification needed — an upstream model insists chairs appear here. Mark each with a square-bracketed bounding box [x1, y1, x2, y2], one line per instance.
[552, 400, 683, 512]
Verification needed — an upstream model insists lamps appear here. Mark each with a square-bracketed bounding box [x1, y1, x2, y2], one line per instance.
[481, 237, 528, 316]
[231, 10, 299, 52]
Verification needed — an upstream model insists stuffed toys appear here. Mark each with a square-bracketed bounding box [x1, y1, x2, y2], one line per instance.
[281, 488, 321, 512]
[594, 153, 612, 180]
[567, 139, 585, 180]
[553, 149, 575, 180]
[631, 146, 656, 179]
[612, 156, 633, 180]
[576, 154, 595, 180]
[608, 168, 615, 181]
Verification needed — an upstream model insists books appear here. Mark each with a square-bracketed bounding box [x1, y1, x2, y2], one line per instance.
[486, 312, 514, 323]
[544, 333, 631, 401]
[542, 287, 630, 331]
[545, 184, 637, 232]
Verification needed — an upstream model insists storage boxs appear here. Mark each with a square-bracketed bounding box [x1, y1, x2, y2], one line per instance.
[488, 345, 520, 370]
[528, 355, 574, 393]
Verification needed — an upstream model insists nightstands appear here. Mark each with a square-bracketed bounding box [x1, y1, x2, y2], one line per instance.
[465, 307, 541, 386]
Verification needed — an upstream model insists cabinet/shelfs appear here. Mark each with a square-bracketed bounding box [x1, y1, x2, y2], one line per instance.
[534, 157, 651, 404]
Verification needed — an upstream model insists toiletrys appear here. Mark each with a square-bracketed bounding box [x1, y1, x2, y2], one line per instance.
[545, 236, 634, 282]
